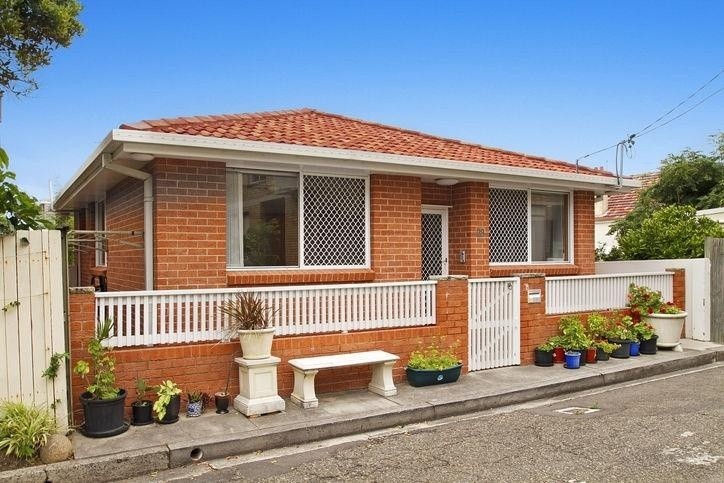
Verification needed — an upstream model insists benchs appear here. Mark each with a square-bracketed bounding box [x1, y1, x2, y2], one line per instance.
[287, 350, 401, 408]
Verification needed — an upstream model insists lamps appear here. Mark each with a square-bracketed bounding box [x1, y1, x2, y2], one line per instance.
[433, 176, 459, 186]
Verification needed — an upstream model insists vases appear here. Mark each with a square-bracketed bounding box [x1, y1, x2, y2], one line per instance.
[640, 308, 688, 347]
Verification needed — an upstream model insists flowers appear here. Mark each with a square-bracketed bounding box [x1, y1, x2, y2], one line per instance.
[620, 283, 681, 324]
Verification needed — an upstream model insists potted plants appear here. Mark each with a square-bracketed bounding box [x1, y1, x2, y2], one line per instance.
[531, 316, 660, 372]
[217, 290, 277, 359]
[71, 322, 230, 442]
[404, 343, 464, 386]
